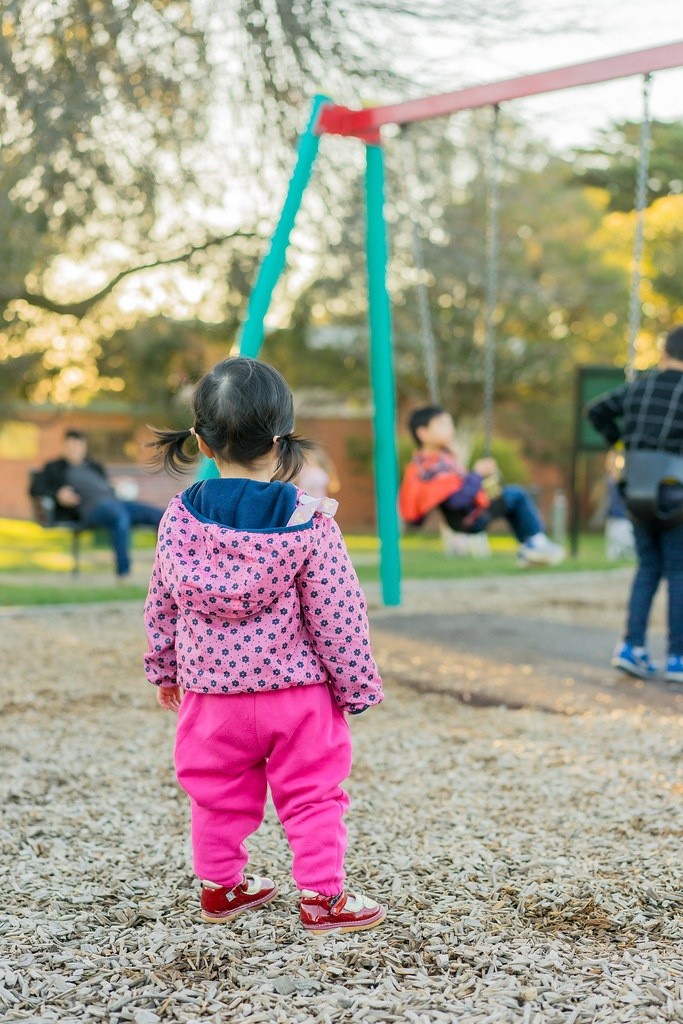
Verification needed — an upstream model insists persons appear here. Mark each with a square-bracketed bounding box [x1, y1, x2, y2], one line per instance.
[583, 324, 683, 683]
[28, 429, 165, 586]
[144, 356, 386, 932]
[397, 404, 567, 569]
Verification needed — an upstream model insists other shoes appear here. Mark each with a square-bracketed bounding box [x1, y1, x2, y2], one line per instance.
[518, 545, 565, 572]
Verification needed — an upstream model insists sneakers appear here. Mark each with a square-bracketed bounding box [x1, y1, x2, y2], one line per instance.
[610, 641, 659, 681]
[662, 654, 683, 683]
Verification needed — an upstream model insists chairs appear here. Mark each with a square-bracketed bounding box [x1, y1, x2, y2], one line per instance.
[27, 469, 95, 587]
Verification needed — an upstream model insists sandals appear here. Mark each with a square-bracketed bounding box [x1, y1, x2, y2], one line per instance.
[298, 888, 387, 935]
[200, 873, 280, 924]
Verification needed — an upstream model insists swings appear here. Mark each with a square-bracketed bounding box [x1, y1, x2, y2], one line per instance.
[389, 104, 505, 532]
[620, 74, 683, 532]
